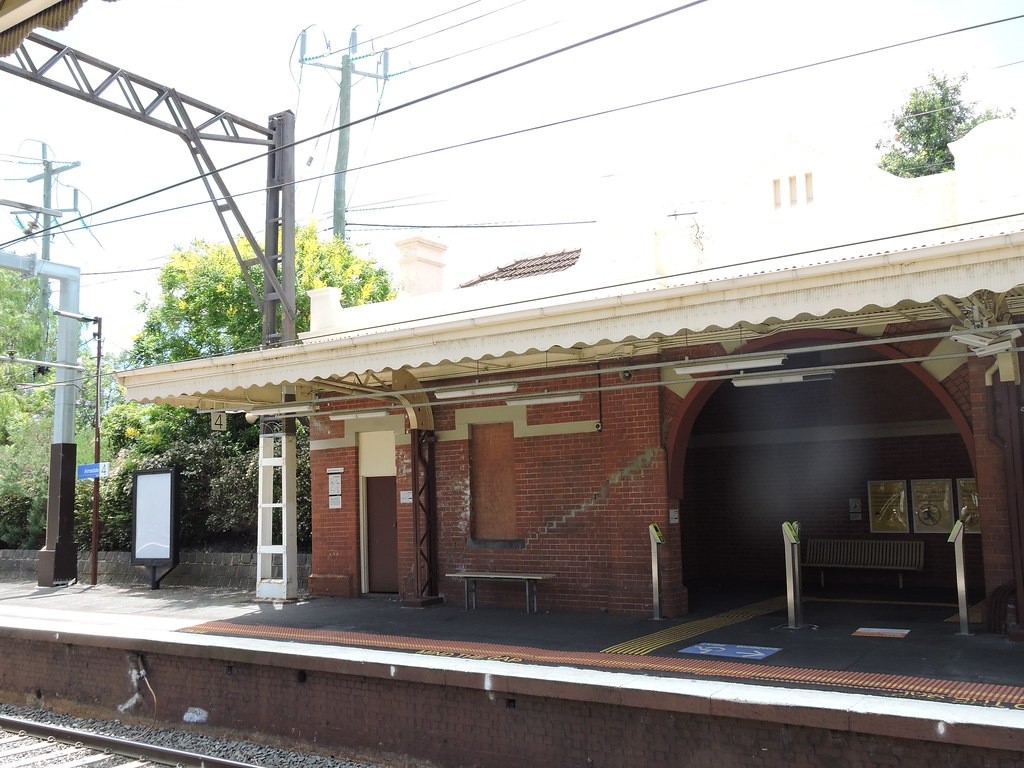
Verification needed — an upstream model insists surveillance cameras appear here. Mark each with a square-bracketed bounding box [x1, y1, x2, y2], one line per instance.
[948, 334, 1013, 358]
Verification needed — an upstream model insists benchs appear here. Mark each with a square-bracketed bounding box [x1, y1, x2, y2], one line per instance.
[801, 537, 925, 590]
[446, 571, 554, 615]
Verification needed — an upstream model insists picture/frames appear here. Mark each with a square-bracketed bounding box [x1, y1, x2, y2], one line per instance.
[867, 480, 910, 534]
[956, 478, 981, 534]
[909, 478, 955, 533]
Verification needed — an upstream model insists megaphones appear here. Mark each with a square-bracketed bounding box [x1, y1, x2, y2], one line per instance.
[619, 370, 636, 382]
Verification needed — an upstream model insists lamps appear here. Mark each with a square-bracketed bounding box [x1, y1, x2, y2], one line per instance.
[672, 354, 788, 375]
[434, 382, 520, 399]
[252, 403, 316, 416]
[330, 408, 389, 420]
